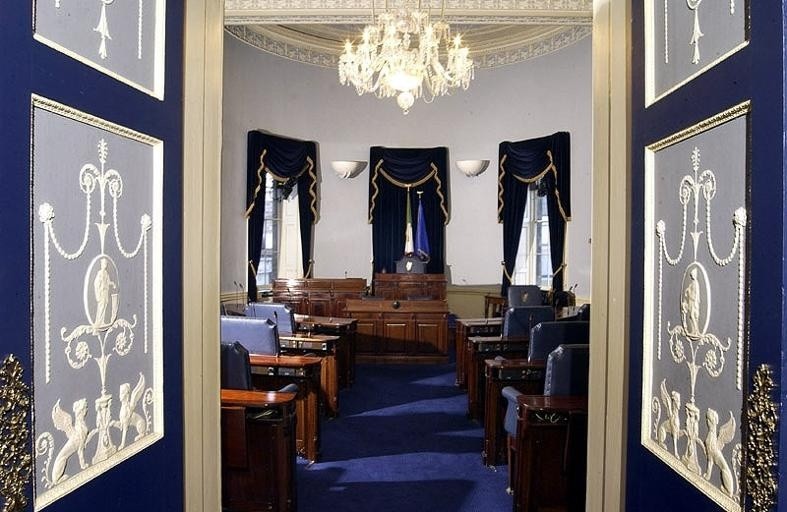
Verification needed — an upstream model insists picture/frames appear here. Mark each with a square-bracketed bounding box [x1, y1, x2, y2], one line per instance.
[31, 0, 164, 102]
[643, 1, 752, 113]
[29, 95, 164, 511]
[640, 99, 753, 511]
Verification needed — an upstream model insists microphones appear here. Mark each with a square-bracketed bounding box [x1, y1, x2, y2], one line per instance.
[221, 280, 334, 363]
[485, 284, 577, 339]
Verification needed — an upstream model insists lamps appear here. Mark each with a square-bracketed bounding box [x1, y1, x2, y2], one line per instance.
[331, 159, 491, 180]
[337, 8, 475, 116]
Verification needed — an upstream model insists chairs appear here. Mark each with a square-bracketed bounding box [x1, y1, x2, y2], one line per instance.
[396, 252, 429, 275]
[484, 283, 556, 339]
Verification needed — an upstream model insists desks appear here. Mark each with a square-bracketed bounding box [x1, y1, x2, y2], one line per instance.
[454, 317, 587, 512]
[220, 303, 358, 510]
